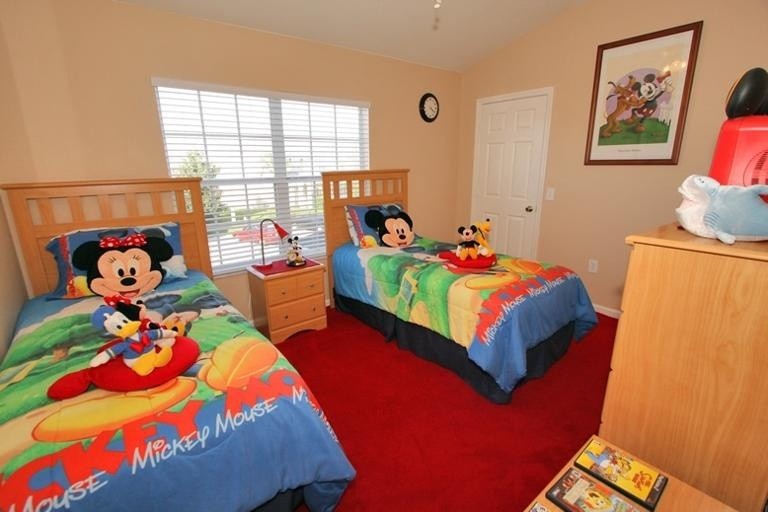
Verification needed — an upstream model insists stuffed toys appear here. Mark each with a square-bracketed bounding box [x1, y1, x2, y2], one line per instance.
[456, 225, 480, 260]
[286, 236, 302, 265]
[676, 173, 768, 245]
[89, 305, 178, 376]
[473, 221, 494, 257]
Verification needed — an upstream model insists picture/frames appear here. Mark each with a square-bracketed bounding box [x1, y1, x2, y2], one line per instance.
[585, 20, 703, 165]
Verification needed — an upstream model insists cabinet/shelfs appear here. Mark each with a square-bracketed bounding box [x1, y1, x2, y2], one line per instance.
[524, 231, 768, 512]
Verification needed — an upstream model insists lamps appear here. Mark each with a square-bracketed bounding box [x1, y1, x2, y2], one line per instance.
[252, 218, 289, 267]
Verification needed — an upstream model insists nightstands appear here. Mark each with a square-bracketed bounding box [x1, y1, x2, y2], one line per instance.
[246, 253, 327, 345]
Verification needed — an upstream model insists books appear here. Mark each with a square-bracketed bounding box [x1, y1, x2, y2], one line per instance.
[529, 438, 668, 512]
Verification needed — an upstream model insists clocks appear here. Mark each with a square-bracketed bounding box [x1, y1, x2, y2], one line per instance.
[419, 92, 439, 122]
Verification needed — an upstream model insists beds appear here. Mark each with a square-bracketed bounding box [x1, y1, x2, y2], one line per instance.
[322, 168, 600, 406]
[1, 177, 357, 512]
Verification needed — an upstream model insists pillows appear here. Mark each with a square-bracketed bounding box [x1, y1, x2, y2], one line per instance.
[344, 201, 418, 249]
[44, 220, 189, 301]
